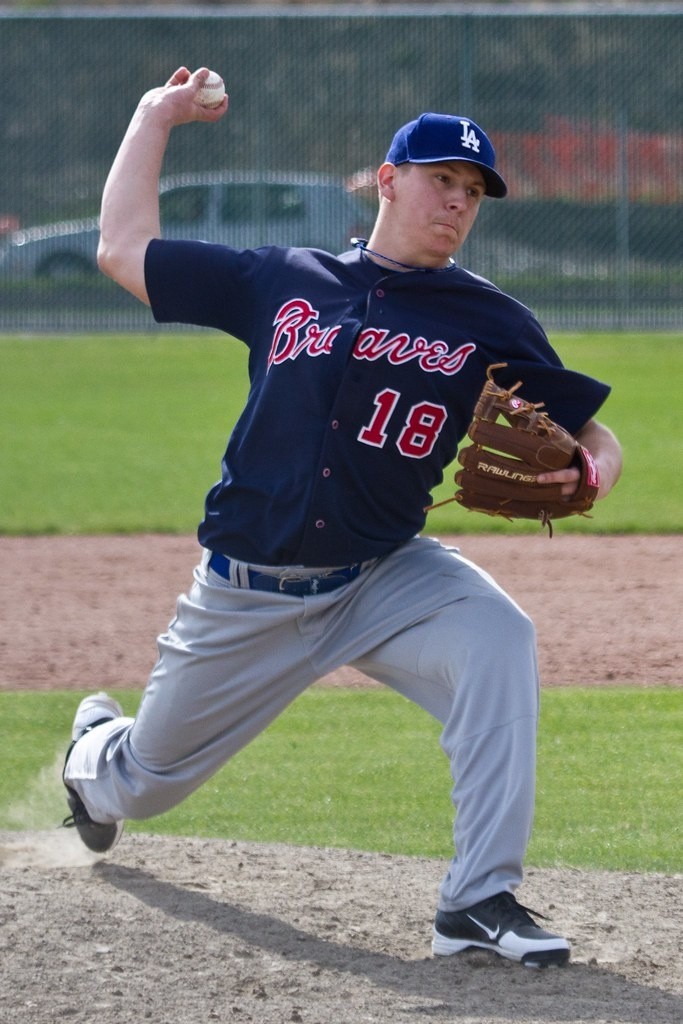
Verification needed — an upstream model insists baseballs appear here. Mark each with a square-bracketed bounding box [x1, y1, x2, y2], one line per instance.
[188, 70, 226, 110]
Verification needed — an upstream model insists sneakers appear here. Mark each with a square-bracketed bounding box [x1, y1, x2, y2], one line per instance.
[430, 889, 570, 970]
[62, 694, 126, 854]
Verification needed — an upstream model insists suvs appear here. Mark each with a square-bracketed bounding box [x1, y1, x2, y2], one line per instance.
[0, 168, 374, 306]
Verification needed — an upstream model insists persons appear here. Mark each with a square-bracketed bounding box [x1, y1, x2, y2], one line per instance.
[59, 66, 626, 964]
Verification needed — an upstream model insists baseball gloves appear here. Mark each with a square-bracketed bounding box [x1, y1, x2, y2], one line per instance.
[453, 379, 600, 522]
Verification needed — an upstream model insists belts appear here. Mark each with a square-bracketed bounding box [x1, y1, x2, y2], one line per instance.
[207, 551, 382, 597]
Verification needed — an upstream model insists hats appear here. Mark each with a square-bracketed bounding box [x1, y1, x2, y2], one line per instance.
[383, 112, 508, 198]
